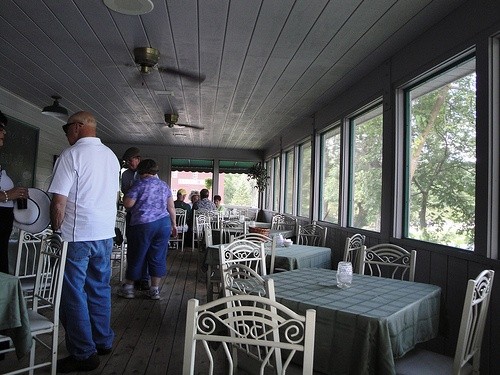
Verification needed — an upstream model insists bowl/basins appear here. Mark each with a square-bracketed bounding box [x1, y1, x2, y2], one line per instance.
[285, 241, 293, 246]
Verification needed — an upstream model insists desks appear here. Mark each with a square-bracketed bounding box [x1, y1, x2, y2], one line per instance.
[211, 228, 294, 246]
[205, 239, 332, 303]
[0, 272, 33, 360]
[231, 267, 441, 375]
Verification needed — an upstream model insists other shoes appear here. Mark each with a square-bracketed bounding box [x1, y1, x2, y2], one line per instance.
[55, 355, 102, 372]
[97, 344, 113, 356]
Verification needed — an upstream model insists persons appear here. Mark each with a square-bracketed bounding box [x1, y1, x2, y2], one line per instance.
[0, 110, 28, 273]
[121, 147, 156, 290]
[174, 189, 192, 246]
[192, 189, 220, 222]
[189, 191, 200, 211]
[213, 195, 228, 218]
[47, 111, 121, 370]
[112, 158, 178, 300]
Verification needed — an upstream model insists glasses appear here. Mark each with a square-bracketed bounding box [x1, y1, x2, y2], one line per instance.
[61, 122, 83, 132]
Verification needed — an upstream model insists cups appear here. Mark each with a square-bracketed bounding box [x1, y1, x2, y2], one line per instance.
[240, 215, 244, 223]
[276, 235, 283, 247]
[337, 262, 352, 288]
[16, 187, 28, 209]
[250, 221, 255, 227]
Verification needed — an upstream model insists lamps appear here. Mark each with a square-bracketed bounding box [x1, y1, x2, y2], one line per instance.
[41, 96, 69, 117]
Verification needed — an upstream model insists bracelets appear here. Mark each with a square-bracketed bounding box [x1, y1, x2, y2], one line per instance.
[2, 190, 8, 203]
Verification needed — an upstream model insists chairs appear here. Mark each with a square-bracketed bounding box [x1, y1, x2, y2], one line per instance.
[343, 233, 366, 273]
[0, 207, 127, 375]
[359, 243, 417, 281]
[167, 208, 328, 374]
[392, 270, 495, 374]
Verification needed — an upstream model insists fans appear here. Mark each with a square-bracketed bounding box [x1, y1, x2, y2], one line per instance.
[137, 112, 205, 132]
[109, 47, 206, 87]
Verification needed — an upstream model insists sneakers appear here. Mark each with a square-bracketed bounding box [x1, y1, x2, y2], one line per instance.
[117, 283, 135, 299]
[146, 288, 161, 299]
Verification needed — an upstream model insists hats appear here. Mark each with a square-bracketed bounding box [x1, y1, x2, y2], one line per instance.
[188, 190, 200, 200]
[177, 189, 188, 194]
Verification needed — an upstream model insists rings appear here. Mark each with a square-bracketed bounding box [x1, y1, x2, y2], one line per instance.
[22, 193, 23, 196]
